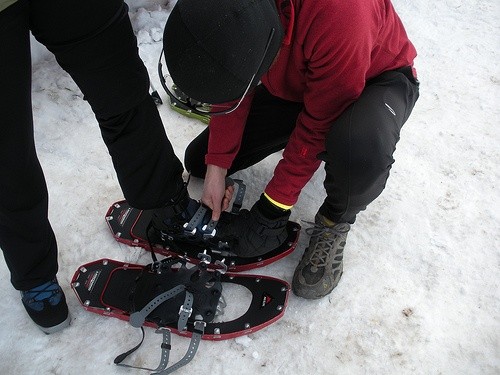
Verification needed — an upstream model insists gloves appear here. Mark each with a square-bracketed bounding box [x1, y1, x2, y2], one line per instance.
[233, 202, 291, 258]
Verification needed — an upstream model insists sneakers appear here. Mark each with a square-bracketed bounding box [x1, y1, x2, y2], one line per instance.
[20, 279, 71, 334]
[152, 196, 244, 249]
[292, 214, 351, 299]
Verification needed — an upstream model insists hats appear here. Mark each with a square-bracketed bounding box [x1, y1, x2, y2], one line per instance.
[163, 0, 282, 105]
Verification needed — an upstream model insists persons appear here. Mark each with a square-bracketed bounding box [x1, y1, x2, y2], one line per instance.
[157, 1, 420, 299]
[0, 0, 242, 333]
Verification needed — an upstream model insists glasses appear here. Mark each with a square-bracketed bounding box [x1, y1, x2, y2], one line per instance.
[156, 29, 275, 116]
